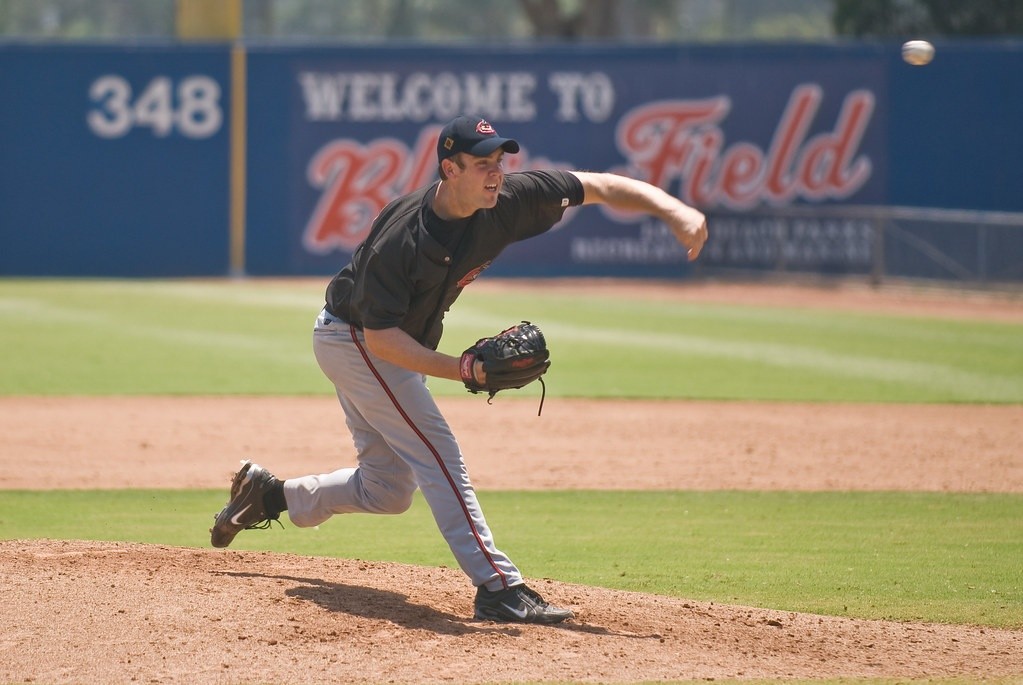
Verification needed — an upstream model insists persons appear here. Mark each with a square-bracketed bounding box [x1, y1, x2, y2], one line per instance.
[209, 116, 708, 625]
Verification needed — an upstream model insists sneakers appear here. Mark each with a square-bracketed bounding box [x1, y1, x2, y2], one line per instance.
[209, 459, 285, 548]
[474, 584, 571, 624]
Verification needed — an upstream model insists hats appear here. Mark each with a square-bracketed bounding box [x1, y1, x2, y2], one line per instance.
[437, 114, 520, 163]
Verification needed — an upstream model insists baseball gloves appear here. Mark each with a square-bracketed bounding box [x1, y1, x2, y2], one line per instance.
[460, 319, 551, 397]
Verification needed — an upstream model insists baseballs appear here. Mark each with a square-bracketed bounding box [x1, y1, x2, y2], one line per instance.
[901, 40, 936, 67]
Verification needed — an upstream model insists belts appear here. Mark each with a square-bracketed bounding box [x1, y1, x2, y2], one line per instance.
[324, 305, 338, 318]
